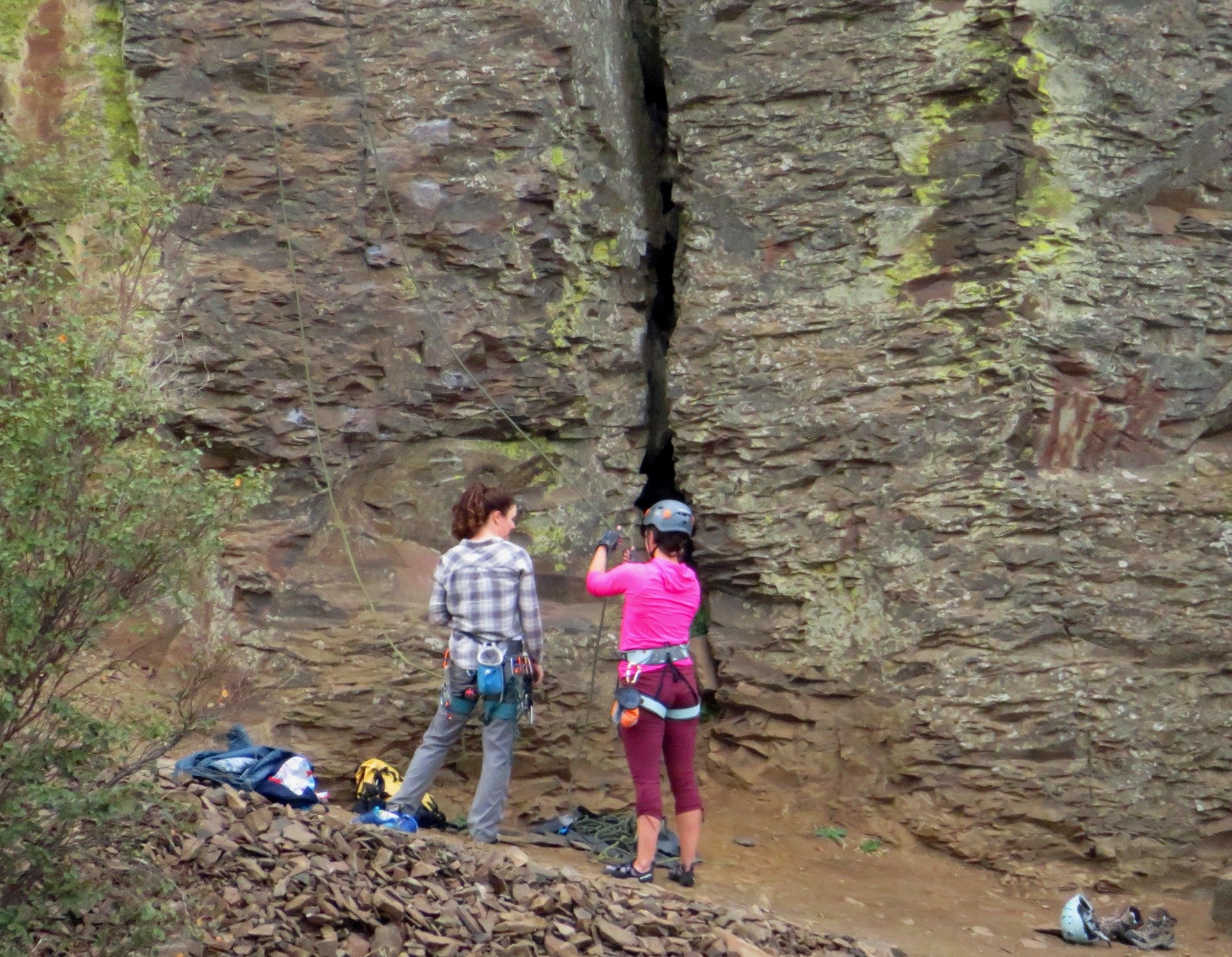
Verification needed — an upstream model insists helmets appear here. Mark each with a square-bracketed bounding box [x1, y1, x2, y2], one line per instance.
[1060, 894, 1113, 949]
[640, 499, 694, 538]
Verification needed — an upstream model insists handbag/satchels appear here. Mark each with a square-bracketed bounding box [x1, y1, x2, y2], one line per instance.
[610, 686, 639, 728]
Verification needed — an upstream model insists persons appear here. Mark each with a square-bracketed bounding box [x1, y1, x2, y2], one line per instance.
[384, 481, 543, 845]
[587, 500, 701, 887]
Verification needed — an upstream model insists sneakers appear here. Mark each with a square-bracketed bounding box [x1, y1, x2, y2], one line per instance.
[1122, 907, 1178, 950]
[602, 858, 656, 883]
[1097, 906, 1143, 945]
[668, 862, 696, 887]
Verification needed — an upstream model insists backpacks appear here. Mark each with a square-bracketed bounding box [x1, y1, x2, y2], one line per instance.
[349, 758, 447, 832]
[172, 745, 329, 807]
